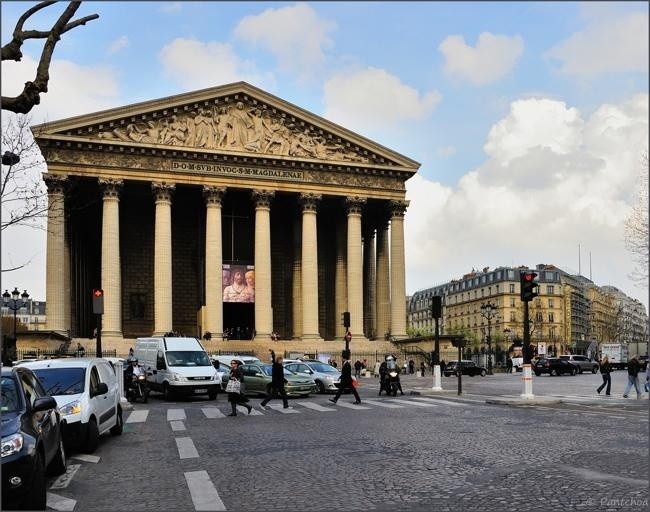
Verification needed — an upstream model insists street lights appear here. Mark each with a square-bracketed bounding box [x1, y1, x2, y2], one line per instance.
[2, 288, 32, 361]
[480, 300, 499, 375]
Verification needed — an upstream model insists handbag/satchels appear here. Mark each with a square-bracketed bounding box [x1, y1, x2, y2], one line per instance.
[225, 380, 241, 394]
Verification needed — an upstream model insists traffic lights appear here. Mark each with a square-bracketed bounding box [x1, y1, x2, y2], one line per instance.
[519, 270, 537, 302]
[451, 339, 458, 346]
[485, 335, 489, 344]
[340, 312, 350, 326]
[93, 289, 104, 313]
[461, 340, 467, 347]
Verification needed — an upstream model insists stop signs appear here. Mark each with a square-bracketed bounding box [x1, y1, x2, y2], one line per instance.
[346, 331, 353, 343]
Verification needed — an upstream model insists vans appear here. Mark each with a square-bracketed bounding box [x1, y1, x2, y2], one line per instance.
[11, 358, 124, 453]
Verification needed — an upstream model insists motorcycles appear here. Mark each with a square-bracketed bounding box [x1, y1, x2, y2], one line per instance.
[122, 367, 150, 404]
[380, 360, 400, 396]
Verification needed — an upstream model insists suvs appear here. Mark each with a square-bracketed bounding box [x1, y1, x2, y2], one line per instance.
[560, 355, 600, 373]
[0, 364, 67, 512]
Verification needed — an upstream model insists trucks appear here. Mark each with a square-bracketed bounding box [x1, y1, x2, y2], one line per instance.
[601, 342, 628, 370]
[627, 341, 650, 371]
[134, 335, 222, 402]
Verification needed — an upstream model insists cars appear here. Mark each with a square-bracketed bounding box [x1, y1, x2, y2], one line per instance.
[443, 359, 489, 378]
[1, 359, 40, 366]
[208, 352, 358, 398]
[533, 356, 578, 376]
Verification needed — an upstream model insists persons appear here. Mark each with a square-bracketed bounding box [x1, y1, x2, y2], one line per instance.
[354, 354, 427, 397]
[328, 355, 362, 405]
[223, 269, 255, 302]
[59, 344, 67, 358]
[596, 357, 613, 396]
[131, 102, 363, 162]
[506, 357, 513, 374]
[623, 354, 644, 399]
[226, 360, 252, 416]
[259, 356, 293, 411]
[77, 343, 85, 358]
[125, 358, 147, 401]
[128, 347, 135, 358]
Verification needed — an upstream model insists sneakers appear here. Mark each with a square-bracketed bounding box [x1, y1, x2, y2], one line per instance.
[286, 407, 293, 409]
[260, 404, 265, 410]
[353, 402, 361, 404]
[329, 398, 336, 404]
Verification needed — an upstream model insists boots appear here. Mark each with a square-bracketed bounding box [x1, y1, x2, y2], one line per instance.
[228, 404, 236, 416]
[239, 403, 252, 415]
